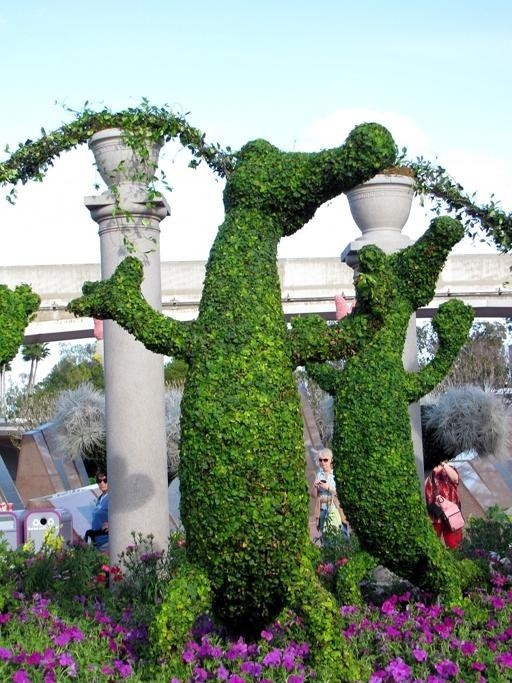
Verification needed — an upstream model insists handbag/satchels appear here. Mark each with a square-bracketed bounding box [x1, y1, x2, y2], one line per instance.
[324, 486, 343, 533]
[430, 472, 466, 532]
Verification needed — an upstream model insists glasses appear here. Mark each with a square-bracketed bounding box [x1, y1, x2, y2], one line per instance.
[95, 478, 108, 484]
[319, 458, 329, 462]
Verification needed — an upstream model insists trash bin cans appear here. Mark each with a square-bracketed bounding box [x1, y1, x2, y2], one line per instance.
[0, 506, 73, 553]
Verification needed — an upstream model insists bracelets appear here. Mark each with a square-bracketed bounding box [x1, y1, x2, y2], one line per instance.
[441, 463, 447, 467]
[313, 484, 317, 488]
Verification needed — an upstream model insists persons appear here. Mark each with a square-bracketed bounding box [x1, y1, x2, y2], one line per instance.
[422, 456, 463, 552]
[89, 469, 112, 555]
[309, 447, 350, 544]
